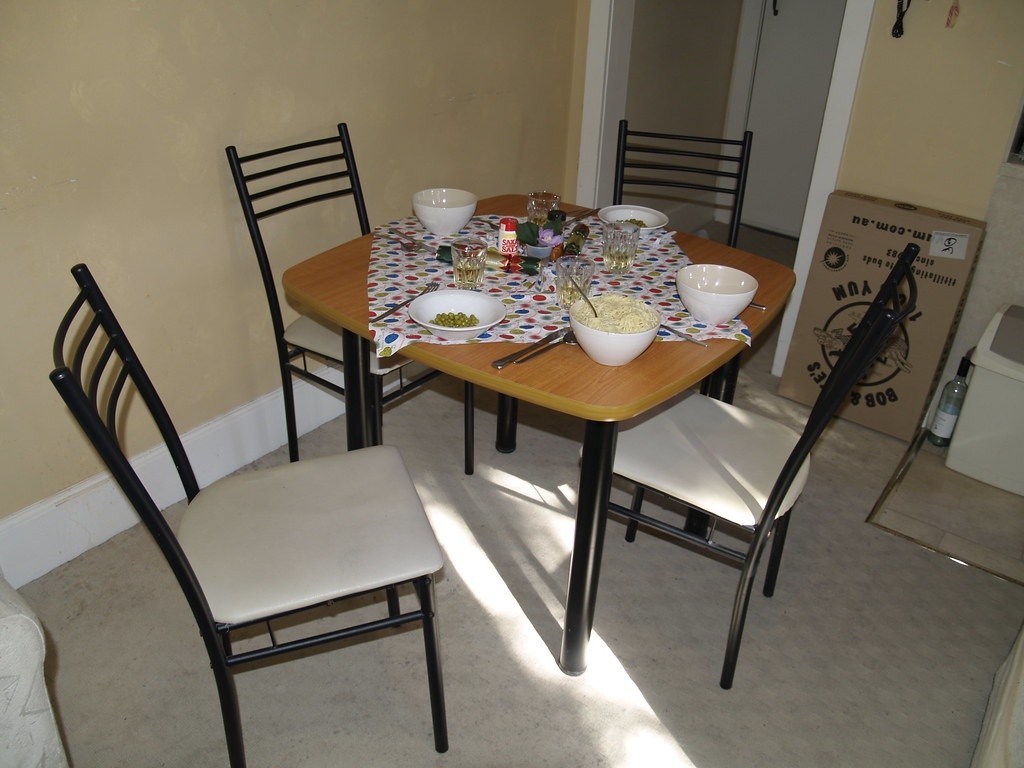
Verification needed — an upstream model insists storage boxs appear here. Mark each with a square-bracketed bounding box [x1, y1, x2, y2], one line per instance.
[774, 190, 987, 436]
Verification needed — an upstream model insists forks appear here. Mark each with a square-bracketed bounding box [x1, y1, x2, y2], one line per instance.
[369, 281, 440, 324]
[372, 233, 422, 252]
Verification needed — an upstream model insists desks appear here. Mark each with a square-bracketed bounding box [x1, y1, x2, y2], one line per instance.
[282, 194, 798, 675]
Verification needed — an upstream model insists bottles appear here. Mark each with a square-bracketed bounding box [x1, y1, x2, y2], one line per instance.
[498, 217, 518, 254]
[928, 356, 970, 447]
[547, 209, 566, 263]
[563, 223, 590, 256]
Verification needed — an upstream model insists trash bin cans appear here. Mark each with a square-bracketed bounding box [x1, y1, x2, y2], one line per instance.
[944, 303, 1024, 496]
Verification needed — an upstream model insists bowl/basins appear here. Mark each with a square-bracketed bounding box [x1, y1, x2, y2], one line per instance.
[408, 289, 506, 340]
[676, 264, 759, 324]
[412, 187, 478, 235]
[570, 296, 661, 367]
[598, 204, 670, 236]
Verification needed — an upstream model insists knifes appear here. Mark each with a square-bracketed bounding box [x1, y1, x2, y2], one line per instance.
[390, 228, 437, 254]
[491, 326, 573, 369]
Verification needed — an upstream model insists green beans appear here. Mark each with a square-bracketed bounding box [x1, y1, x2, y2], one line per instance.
[429, 312, 480, 327]
[616, 219, 648, 228]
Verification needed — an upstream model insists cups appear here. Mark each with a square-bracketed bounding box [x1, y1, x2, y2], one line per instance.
[602, 221, 640, 275]
[528, 188, 561, 226]
[555, 256, 596, 310]
[452, 238, 487, 290]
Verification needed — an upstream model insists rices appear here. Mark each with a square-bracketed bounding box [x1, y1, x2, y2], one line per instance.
[572, 291, 658, 333]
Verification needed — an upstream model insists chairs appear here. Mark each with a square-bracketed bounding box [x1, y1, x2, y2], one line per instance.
[225, 122, 473, 481]
[560, 243, 921, 681]
[52, 262, 447, 768]
[614, 119, 754, 245]
